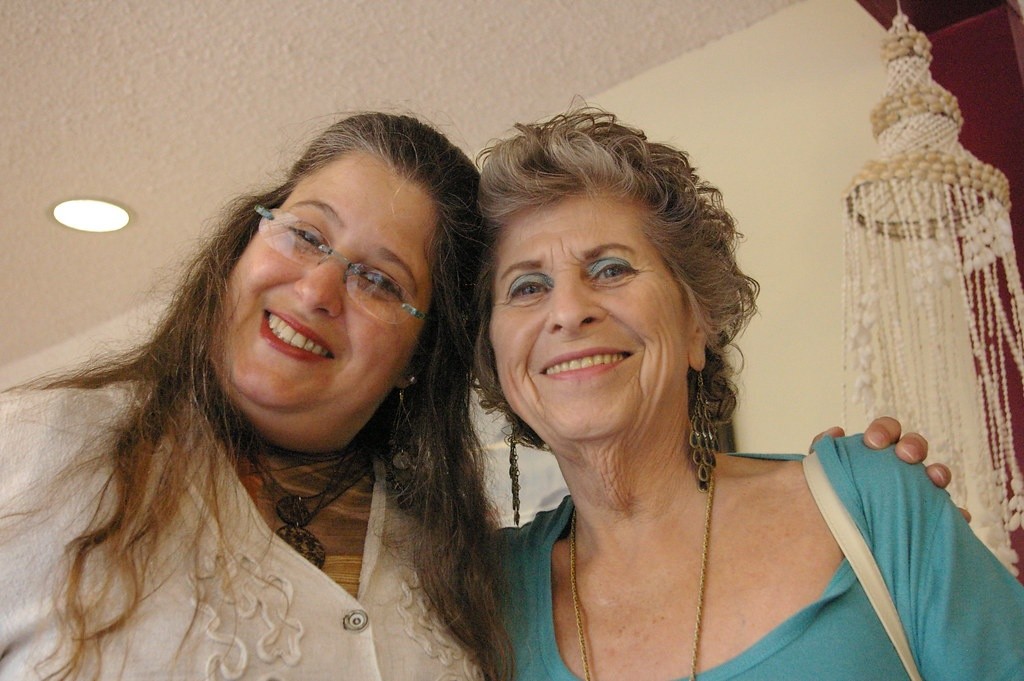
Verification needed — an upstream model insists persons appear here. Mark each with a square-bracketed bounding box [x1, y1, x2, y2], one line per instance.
[457, 95, 1024, 681]
[0, 110, 513, 681]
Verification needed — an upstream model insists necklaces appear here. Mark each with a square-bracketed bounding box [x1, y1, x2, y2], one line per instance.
[233, 436, 373, 569]
[570, 458, 716, 681]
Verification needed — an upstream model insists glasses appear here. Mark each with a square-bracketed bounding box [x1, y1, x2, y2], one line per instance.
[254, 203, 438, 323]
